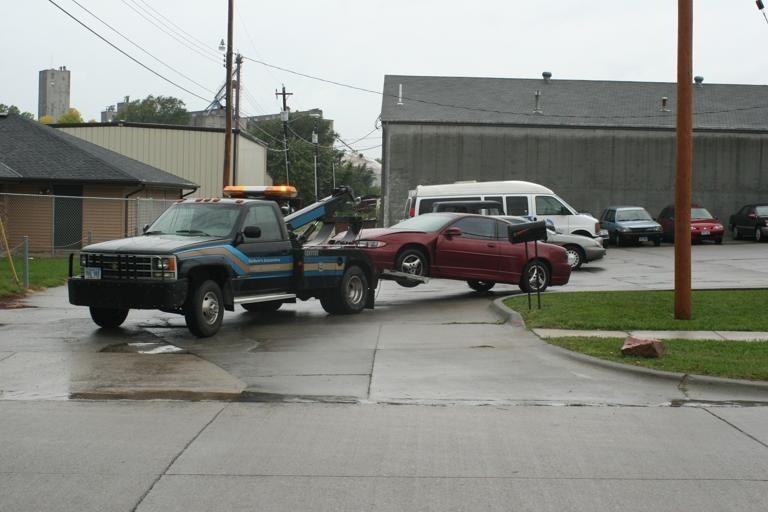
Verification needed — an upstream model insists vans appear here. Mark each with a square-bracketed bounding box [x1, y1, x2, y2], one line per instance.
[409, 180, 610, 248]
[403, 189, 417, 221]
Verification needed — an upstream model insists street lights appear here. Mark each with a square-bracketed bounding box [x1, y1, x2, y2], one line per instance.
[280, 109, 292, 188]
[755, 0, 768, 24]
[311, 132, 319, 203]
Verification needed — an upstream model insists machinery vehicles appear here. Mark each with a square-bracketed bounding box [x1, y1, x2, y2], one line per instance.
[64, 182, 343, 344]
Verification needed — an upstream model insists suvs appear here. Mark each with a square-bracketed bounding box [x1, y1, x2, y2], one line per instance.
[432, 198, 557, 233]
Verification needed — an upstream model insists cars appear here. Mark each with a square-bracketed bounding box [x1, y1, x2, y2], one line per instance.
[488, 213, 607, 270]
[728, 201, 768, 244]
[325, 208, 572, 296]
[598, 205, 665, 248]
[653, 202, 725, 246]
